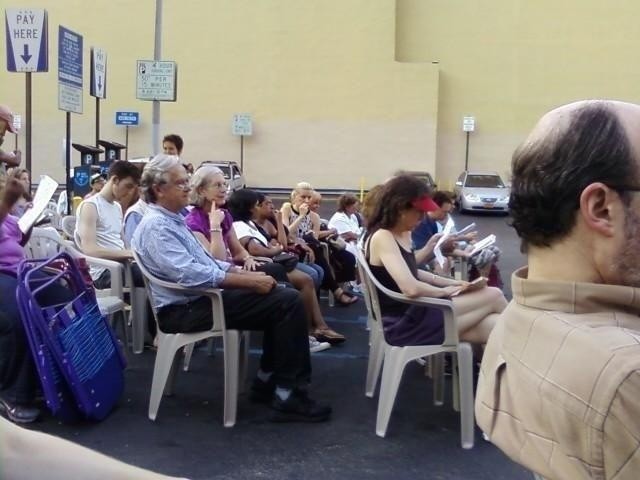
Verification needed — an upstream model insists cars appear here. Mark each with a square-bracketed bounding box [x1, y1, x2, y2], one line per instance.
[126, 156, 247, 206]
[394, 167, 438, 200]
[454, 170, 512, 215]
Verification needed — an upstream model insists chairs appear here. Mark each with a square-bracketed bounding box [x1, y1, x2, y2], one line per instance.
[482, 432, 547, 479]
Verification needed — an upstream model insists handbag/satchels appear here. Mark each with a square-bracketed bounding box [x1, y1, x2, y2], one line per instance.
[272, 248, 298, 274]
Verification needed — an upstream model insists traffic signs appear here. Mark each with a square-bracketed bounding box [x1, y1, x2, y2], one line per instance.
[56, 24, 84, 88]
[115, 110, 141, 127]
[232, 113, 253, 137]
[57, 82, 85, 115]
[135, 58, 178, 102]
[11, 114, 20, 129]
[90, 45, 108, 99]
[5, 7, 49, 75]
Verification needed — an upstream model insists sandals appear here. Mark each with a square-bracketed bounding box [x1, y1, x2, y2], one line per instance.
[335, 289, 359, 305]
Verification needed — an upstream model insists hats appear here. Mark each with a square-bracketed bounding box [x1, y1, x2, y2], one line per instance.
[89, 172, 107, 188]
[0, 104, 19, 135]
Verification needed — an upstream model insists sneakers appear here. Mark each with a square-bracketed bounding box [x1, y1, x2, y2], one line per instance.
[0, 388, 42, 424]
[308, 325, 347, 353]
[245, 374, 332, 421]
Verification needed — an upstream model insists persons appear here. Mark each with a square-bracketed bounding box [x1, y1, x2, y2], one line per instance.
[474, 100, 640, 480]
[0, 106, 507, 427]
